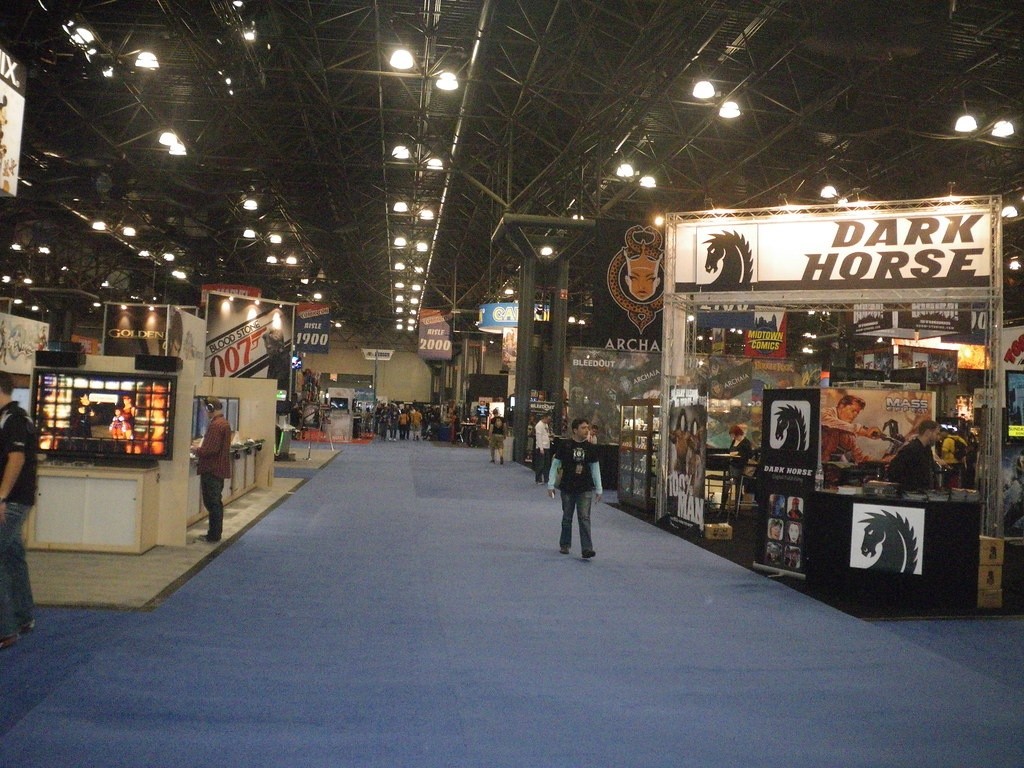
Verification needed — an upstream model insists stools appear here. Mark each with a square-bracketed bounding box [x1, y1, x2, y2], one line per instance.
[734, 462, 759, 519]
[704, 456, 733, 515]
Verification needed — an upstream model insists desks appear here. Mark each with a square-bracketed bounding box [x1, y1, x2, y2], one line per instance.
[814, 488, 982, 616]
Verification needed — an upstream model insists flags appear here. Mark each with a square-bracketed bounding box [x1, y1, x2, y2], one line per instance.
[293, 300, 331, 354]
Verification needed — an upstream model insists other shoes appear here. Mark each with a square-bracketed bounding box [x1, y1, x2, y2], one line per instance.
[200, 534, 218, 543]
[582, 551, 596, 558]
[500, 456, 503, 464]
[537, 480, 542, 485]
[560, 546, 569, 554]
[382, 435, 419, 441]
[490, 460, 495, 463]
[0, 620, 36, 648]
[544, 480, 549, 484]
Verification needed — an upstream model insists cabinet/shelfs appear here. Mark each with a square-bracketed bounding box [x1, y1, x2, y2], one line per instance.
[22, 460, 160, 556]
[618, 399, 665, 517]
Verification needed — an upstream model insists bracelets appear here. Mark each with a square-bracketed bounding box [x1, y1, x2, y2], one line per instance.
[0, 497, 7, 503]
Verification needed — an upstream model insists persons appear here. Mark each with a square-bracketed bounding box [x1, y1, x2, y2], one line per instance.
[506, 328, 515, 356]
[476, 400, 490, 416]
[488, 408, 507, 465]
[189, 395, 233, 544]
[821, 394, 883, 464]
[716, 425, 753, 520]
[535, 413, 552, 485]
[936, 425, 978, 489]
[0, 370, 40, 648]
[668, 409, 701, 477]
[768, 493, 802, 569]
[547, 418, 603, 558]
[449, 411, 458, 444]
[1003, 450, 1024, 502]
[587, 425, 599, 445]
[886, 420, 942, 493]
[364, 402, 440, 441]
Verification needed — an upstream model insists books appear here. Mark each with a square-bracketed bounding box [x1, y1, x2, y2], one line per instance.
[865, 479, 979, 501]
